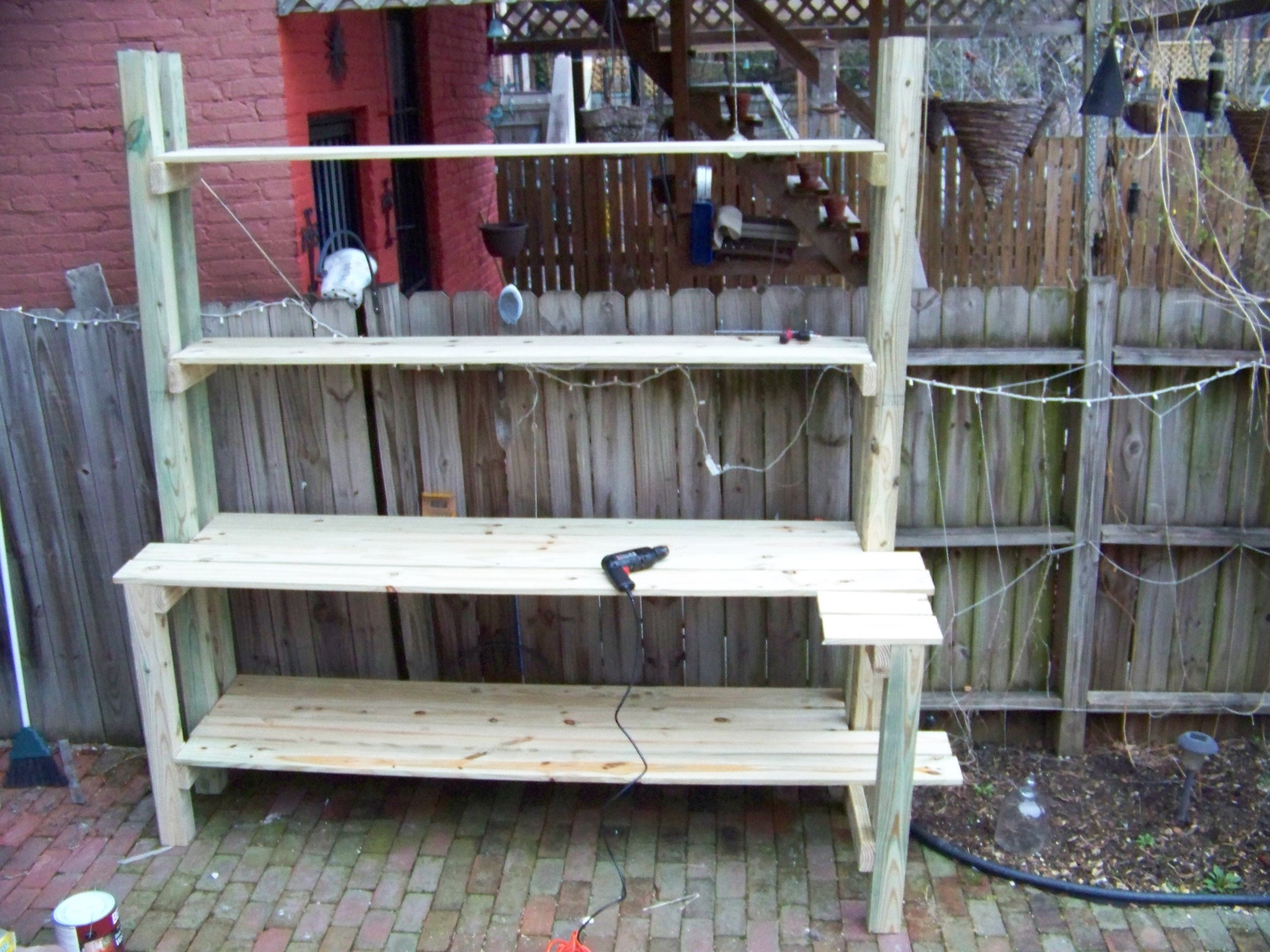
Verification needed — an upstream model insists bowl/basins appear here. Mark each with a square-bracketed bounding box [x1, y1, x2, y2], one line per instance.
[1175, 77, 1209, 113]
[479, 222, 529, 259]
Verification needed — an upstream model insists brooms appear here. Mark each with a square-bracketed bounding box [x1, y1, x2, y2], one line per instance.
[0, 506, 73, 790]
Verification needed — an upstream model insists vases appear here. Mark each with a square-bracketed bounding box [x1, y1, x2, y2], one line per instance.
[854, 232, 870, 252]
[476, 221, 529, 258]
[820, 195, 851, 223]
[725, 92, 751, 117]
[1176, 78, 1226, 115]
[794, 162, 823, 192]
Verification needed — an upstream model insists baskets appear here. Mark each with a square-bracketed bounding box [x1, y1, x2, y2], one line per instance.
[577, 106, 648, 143]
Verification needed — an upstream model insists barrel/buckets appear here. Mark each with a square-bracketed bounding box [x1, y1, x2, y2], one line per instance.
[52, 881, 123, 951]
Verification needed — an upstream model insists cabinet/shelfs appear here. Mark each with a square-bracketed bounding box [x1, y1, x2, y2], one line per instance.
[107, 35, 967, 938]
